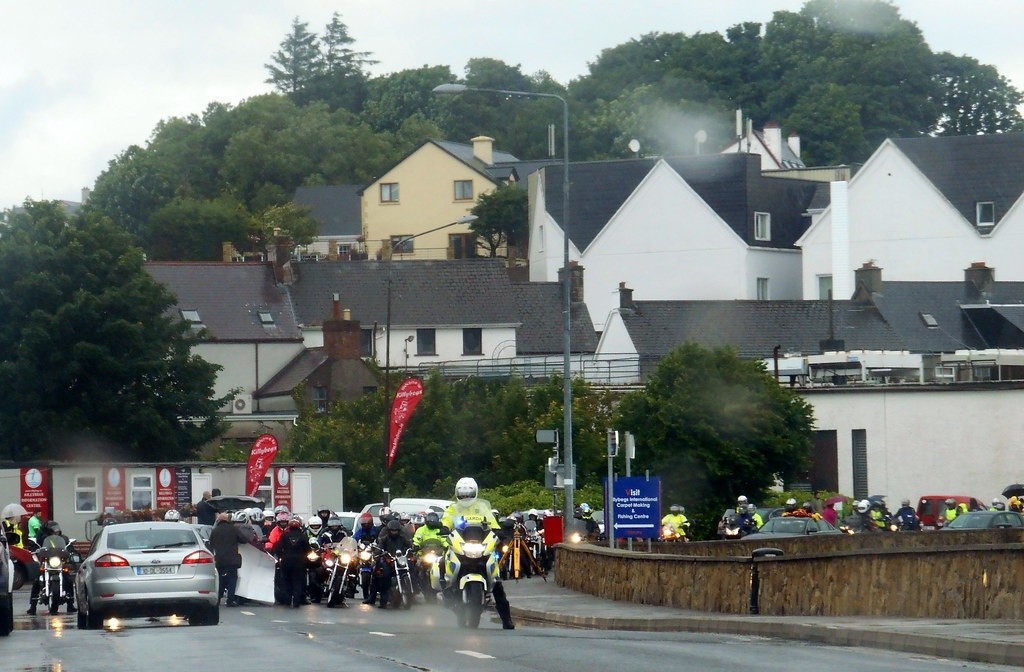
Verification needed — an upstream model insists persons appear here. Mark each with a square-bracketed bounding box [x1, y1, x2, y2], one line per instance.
[2, 511, 17, 532]
[197, 491, 216, 525]
[938, 498, 957, 526]
[228, 505, 312, 607]
[782, 490, 838, 527]
[850, 498, 921, 532]
[209, 512, 247, 606]
[27, 520, 77, 615]
[1008, 496, 1024, 516]
[353, 506, 448, 604]
[439, 477, 515, 630]
[29, 508, 42, 536]
[736, 495, 763, 537]
[990, 497, 1005, 511]
[164, 509, 180, 521]
[305, 503, 359, 604]
[956, 503, 968, 515]
[661, 504, 694, 542]
[490, 502, 600, 581]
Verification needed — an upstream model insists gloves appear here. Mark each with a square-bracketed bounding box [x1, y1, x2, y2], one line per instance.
[440, 526, 450, 536]
[495, 531, 506, 540]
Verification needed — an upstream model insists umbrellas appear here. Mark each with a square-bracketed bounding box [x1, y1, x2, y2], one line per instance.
[1001, 480, 1024, 500]
[1, 503, 28, 532]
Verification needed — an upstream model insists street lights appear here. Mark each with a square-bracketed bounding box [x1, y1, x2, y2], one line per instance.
[433, 83, 572, 533]
[382, 215, 478, 508]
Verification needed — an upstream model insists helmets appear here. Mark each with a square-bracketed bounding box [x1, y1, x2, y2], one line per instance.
[456, 477, 478, 506]
[103, 519, 117, 528]
[491, 502, 592, 526]
[379, 507, 427, 532]
[425, 512, 439, 529]
[164, 510, 180, 520]
[222, 506, 303, 529]
[46, 521, 61, 537]
[737, 490, 1024, 514]
[309, 515, 323, 534]
[670, 505, 685, 515]
[317, 506, 343, 528]
[360, 514, 374, 529]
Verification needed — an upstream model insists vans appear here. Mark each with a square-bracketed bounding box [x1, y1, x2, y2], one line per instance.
[917, 496, 987, 528]
[390, 498, 454, 520]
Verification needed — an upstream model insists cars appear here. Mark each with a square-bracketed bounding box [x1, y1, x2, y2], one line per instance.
[939, 512, 1024, 528]
[8, 544, 40, 591]
[74, 520, 219, 630]
[742, 516, 841, 540]
[191, 524, 213, 548]
[0, 521, 20, 637]
[755, 508, 785, 524]
[308, 503, 383, 536]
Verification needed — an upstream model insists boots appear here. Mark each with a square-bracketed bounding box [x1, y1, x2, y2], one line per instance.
[494, 601, 514, 629]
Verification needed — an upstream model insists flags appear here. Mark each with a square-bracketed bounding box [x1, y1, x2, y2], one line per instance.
[245, 433, 279, 496]
[388, 377, 424, 468]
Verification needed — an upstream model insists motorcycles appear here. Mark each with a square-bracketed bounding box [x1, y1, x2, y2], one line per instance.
[26, 538, 77, 615]
[425, 512, 515, 629]
[305, 518, 590, 609]
[840, 514, 922, 533]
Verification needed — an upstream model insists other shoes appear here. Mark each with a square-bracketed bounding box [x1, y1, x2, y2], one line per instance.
[26, 604, 37, 614]
[66, 604, 77, 611]
[347, 586, 389, 609]
[224, 602, 237, 607]
[277, 589, 321, 607]
